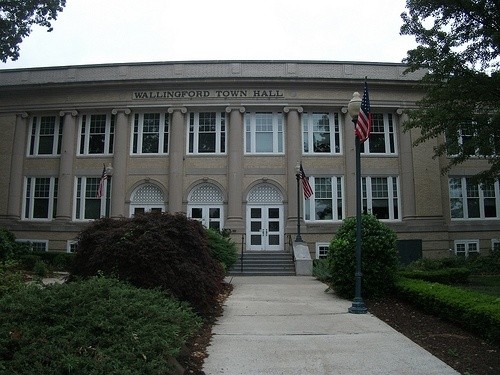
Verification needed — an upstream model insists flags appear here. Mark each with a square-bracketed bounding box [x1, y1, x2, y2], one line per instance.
[355, 80, 373, 143]
[300, 165, 314, 200]
[97, 165, 108, 197]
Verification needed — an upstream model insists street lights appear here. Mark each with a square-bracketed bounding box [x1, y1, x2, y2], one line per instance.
[347, 91, 368, 314]
[294, 161, 304, 242]
[105, 162, 114, 218]
